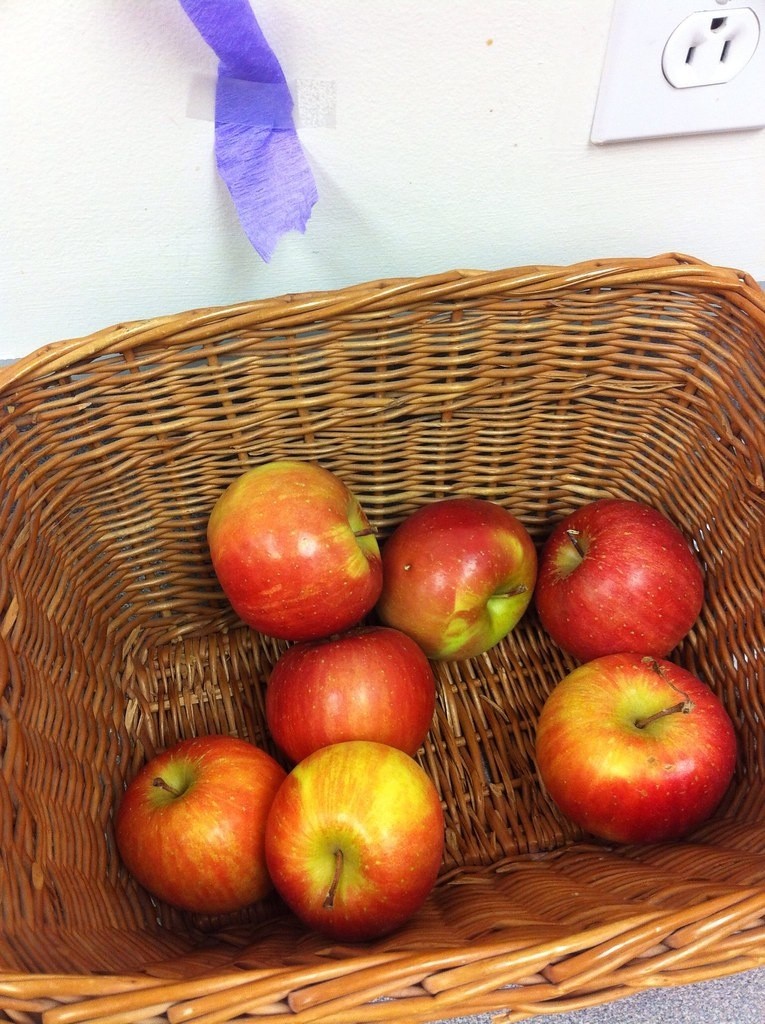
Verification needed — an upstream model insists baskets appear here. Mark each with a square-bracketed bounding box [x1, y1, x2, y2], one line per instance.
[0, 256, 764, 1024]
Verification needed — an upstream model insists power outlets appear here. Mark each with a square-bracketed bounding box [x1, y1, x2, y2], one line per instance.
[590, 3, 765, 144]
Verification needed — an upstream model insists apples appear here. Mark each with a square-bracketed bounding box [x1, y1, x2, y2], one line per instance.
[264, 741, 444, 945]
[265, 626, 435, 766]
[534, 652, 738, 844]
[373, 499, 536, 661]
[205, 461, 383, 642]
[534, 496, 705, 662]
[114, 734, 290, 914]
